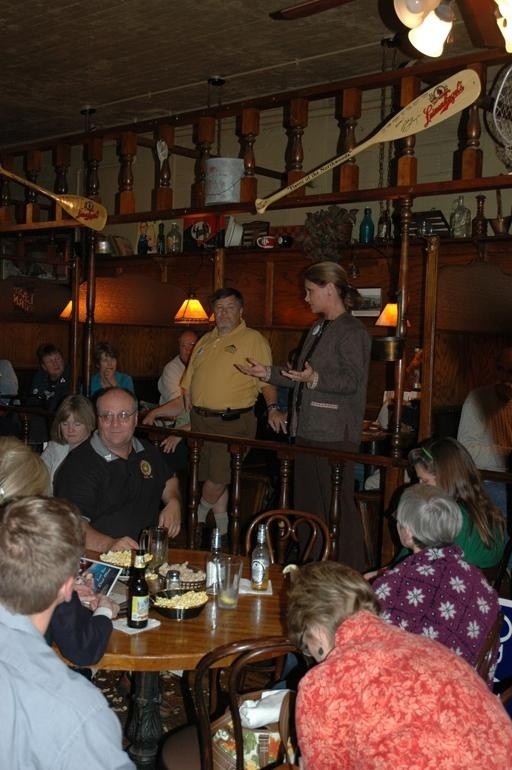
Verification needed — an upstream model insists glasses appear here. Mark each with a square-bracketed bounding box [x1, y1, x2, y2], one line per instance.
[181, 343, 195, 348]
[98, 411, 136, 423]
[298, 629, 310, 657]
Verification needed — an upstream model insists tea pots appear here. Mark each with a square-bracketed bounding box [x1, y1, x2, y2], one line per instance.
[256, 236, 276, 249]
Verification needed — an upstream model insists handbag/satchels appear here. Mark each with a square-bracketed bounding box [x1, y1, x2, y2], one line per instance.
[209, 688, 298, 770]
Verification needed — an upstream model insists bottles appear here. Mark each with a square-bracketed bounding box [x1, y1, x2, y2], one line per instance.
[206, 529, 227, 595]
[450, 194, 472, 239]
[376, 200, 392, 241]
[165, 570, 180, 590]
[359, 207, 374, 244]
[156, 223, 165, 256]
[127, 550, 149, 628]
[472, 195, 488, 238]
[125, 550, 137, 576]
[252, 524, 270, 592]
[165, 223, 181, 255]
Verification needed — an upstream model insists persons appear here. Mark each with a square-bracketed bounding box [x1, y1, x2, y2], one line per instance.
[456, 339, 511, 527]
[1, 495, 142, 768]
[363, 480, 502, 671]
[279, 559, 512, 770]
[1, 285, 290, 718]
[230, 257, 374, 575]
[365, 434, 509, 580]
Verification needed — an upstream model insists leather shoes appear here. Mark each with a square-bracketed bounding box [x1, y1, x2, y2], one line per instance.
[194, 506, 205, 550]
[222, 534, 229, 553]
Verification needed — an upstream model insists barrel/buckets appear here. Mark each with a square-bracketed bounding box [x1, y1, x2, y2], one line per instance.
[202, 157, 245, 207]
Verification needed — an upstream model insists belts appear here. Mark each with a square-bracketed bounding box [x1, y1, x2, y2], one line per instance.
[193, 405, 254, 418]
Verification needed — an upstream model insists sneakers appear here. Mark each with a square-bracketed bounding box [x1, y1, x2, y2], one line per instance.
[116, 673, 172, 720]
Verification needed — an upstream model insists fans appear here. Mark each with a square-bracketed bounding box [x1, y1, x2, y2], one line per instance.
[268, 1, 512, 56]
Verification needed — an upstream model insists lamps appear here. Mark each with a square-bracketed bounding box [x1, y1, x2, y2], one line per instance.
[172, 270, 210, 326]
[372, 287, 412, 330]
[391, 0, 460, 59]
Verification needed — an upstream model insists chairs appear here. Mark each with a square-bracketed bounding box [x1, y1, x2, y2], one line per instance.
[205, 507, 334, 717]
[158, 630, 306, 770]
[471, 611, 504, 683]
[492, 536, 511, 594]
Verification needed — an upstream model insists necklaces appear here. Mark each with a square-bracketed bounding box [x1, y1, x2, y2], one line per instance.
[317, 316, 335, 337]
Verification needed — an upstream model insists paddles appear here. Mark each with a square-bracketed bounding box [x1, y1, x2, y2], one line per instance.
[254, 70, 482, 213]
[0, 167, 108, 230]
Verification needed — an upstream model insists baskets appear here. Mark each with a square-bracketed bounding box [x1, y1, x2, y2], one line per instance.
[155, 562, 206, 590]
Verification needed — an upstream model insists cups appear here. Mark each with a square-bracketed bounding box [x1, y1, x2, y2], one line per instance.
[139, 527, 169, 568]
[215, 557, 243, 610]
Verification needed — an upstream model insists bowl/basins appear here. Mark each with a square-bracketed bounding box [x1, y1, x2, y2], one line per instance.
[150, 589, 207, 620]
